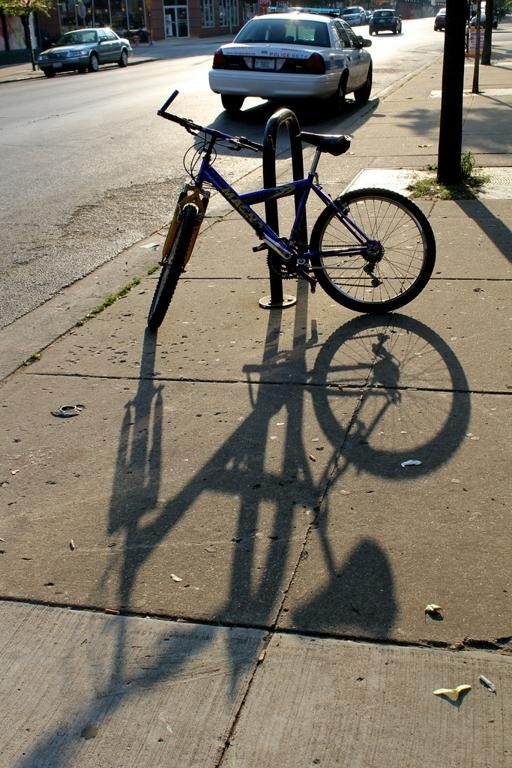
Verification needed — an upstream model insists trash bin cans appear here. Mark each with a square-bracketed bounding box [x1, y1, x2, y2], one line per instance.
[468, 26, 485, 56]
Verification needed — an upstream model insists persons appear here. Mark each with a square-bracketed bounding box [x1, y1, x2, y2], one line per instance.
[469, 10, 477, 23]
[68, 22, 144, 43]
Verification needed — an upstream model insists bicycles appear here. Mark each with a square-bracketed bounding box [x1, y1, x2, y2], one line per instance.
[146, 89, 435, 330]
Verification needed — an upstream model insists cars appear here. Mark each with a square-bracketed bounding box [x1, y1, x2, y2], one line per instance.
[469, 14, 498, 29]
[36, 25, 130, 78]
[208, 6, 373, 115]
[337, 4, 404, 37]
[433, 6, 448, 31]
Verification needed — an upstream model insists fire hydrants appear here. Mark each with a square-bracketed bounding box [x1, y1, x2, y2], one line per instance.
[133, 34, 142, 48]
